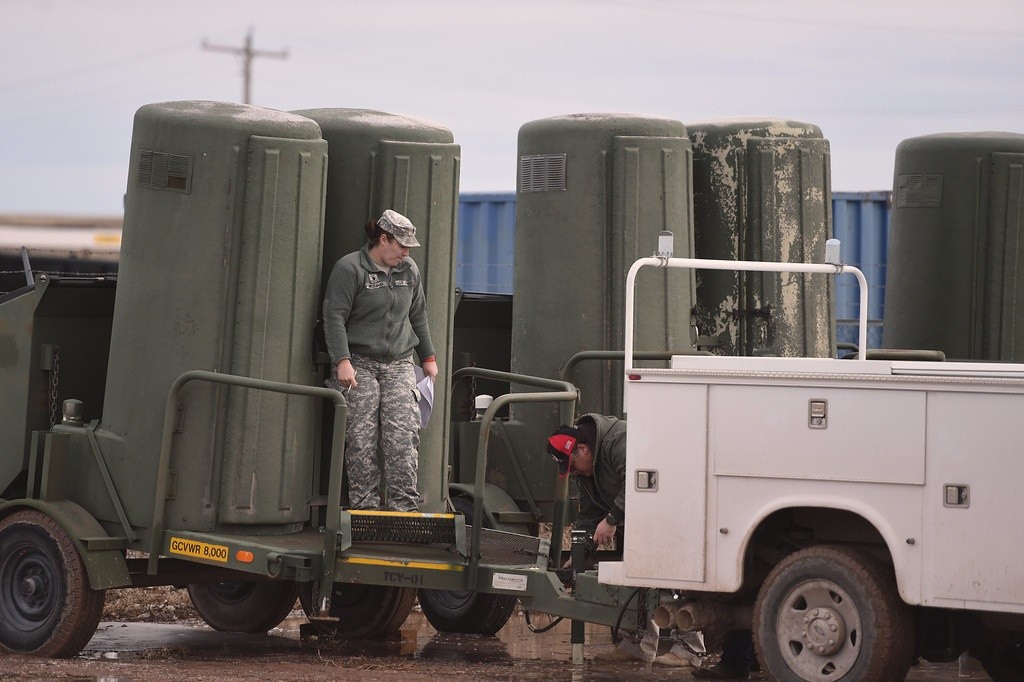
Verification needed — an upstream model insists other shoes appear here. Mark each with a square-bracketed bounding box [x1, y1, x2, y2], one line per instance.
[350, 519, 376, 533]
[390, 520, 433, 538]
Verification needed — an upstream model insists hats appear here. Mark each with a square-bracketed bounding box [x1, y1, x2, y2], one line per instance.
[548, 425, 583, 479]
[377, 209, 421, 248]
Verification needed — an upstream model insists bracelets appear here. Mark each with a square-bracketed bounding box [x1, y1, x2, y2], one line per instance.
[423, 355, 437, 362]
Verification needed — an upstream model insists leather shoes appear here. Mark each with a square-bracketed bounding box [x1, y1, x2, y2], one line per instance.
[595, 648, 641, 663]
[654, 651, 692, 666]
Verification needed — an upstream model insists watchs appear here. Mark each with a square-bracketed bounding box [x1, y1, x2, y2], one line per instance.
[605, 514, 619, 525]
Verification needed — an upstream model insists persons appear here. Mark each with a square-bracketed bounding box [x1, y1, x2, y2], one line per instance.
[546, 413, 625, 569]
[322, 209, 439, 511]
[595, 617, 706, 667]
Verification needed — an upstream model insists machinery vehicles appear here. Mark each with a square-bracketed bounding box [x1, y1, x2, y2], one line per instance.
[0, 98, 1024, 680]
